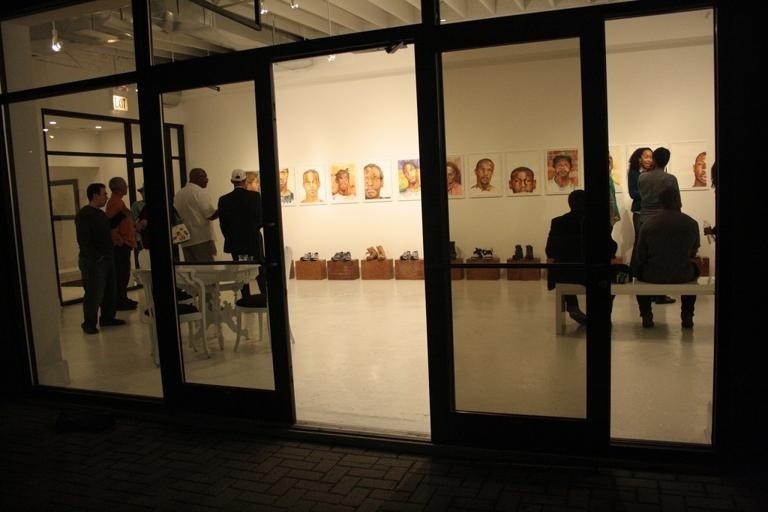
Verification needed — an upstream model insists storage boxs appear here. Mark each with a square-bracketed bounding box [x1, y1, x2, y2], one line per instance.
[290, 258, 553, 281]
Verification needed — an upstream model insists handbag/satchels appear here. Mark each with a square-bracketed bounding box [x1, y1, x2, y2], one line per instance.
[171, 223, 190, 243]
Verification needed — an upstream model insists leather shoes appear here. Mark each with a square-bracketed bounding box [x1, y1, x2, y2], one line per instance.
[84, 299, 137, 334]
[656, 296, 675, 304]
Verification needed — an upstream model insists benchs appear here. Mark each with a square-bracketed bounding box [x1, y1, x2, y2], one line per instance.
[556, 284, 716, 342]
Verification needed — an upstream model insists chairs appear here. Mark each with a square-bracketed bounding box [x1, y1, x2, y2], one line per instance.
[132, 245, 295, 368]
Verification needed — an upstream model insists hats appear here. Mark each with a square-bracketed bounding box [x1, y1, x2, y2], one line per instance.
[232, 169, 246, 181]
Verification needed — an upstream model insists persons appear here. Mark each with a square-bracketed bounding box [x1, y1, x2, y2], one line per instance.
[608, 148, 676, 304]
[218, 170, 268, 308]
[468, 158, 497, 195]
[74, 179, 187, 333]
[364, 165, 391, 202]
[279, 168, 296, 205]
[332, 170, 355, 202]
[503, 167, 541, 192]
[175, 168, 223, 311]
[629, 189, 700, 328]
[547, 190, 622, 327]
[396, 160, 421, 198]
[690, 152, 707, 187]
[301, 170, 326, 203]
[546, 150, 576, 188]
[243, 172, 261, 193]
[445, 158, 463, 197]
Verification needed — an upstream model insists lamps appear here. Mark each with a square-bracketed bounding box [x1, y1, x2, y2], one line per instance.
[50, 22, 64, 53]
